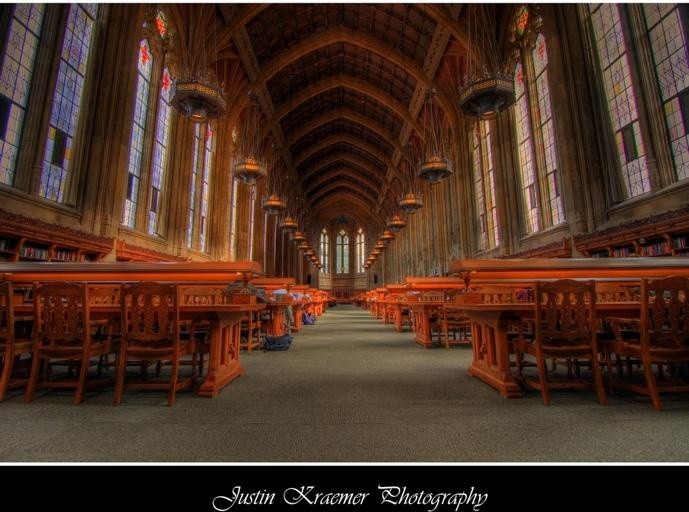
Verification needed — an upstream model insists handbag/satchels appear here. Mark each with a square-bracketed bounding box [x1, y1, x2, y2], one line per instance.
[303, 312, 315, 324]
[263, 334, 292, 351]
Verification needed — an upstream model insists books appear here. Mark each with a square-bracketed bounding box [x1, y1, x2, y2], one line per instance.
[19, 245, 85, 263]
[590, 234, 689, 259]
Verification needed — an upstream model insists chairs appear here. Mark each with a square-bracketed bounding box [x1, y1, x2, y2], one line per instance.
[1, 261, 332, 407]
[349, 257, 689, 410]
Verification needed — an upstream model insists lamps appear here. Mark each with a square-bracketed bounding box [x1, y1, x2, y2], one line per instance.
[361, 3, 516, 268]
[168, 3, 321, 270]
